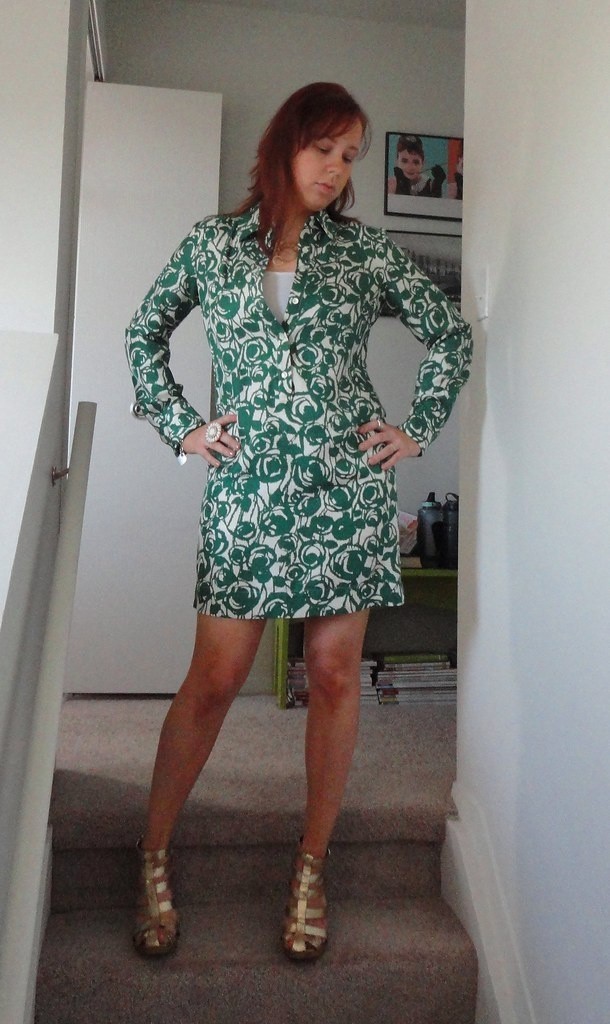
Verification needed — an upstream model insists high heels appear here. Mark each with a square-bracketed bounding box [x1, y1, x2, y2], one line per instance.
[279, 833, 333, 963]
[131, 834, 182, 956]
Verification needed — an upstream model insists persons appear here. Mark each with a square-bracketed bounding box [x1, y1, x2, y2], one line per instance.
[445, 139, 464, 200]
[122, 79, 479, 965]
[387, 135, 447, 199]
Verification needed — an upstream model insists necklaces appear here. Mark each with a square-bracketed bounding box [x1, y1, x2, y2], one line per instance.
[269, 237, 302, 267]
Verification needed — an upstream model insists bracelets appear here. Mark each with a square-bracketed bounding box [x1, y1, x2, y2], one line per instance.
[177, 440, 189, 466]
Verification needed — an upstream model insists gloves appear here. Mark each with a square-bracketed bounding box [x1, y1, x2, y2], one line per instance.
[431, 164, 446, 197]
[454, 173, 463, 200]
[394, 167, 410, 194]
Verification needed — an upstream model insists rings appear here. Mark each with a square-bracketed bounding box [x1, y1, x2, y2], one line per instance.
[375, 419, 385, 431]
[205, 422, 222, 443]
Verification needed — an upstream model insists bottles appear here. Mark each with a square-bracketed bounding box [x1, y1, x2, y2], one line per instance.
[442, 492, 457, 569]
[418, 492, 448, 569]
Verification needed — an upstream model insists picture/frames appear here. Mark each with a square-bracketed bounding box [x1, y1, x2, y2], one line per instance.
[383, 132, 463, 221]
